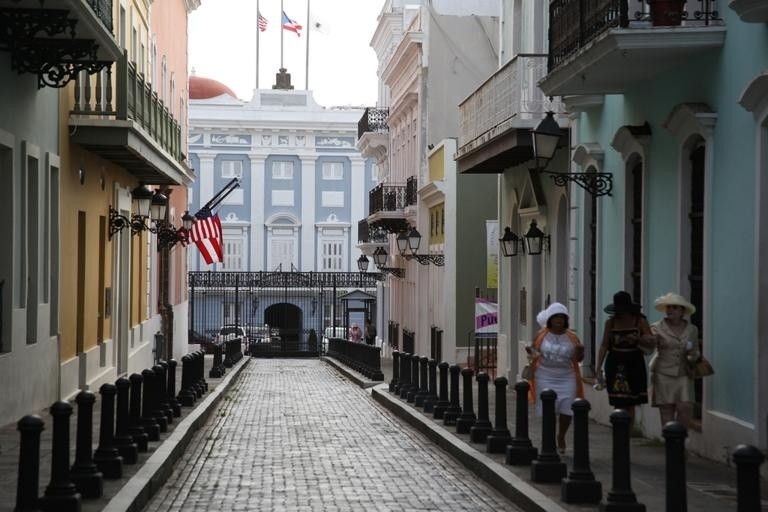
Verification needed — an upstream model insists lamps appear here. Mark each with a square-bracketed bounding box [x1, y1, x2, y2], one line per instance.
[108, 182, 152, 242]
[407, 226, 444, 267]
[497, 227, 524, 257]
[169, 210, 192, 250]
[130, 187, 167, 236]
[396, 231, 429, 266]
[522, 219, 551, 255]
[356, 246, 400, 281]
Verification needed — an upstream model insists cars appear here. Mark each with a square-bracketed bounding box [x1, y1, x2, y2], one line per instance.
[321, 326, 354, 353]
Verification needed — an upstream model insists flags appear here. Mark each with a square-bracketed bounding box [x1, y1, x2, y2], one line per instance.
[177, 206, 218, 247]
[258, 13, 268, 32]
[283, 11, 302, 37]
[191, 213, 225, 265]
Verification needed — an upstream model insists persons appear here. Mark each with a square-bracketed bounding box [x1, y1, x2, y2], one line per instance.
[349, 322, 361, 341]
[523, 301, 585, 453]
[641, 293, 701, 436]
[594, 291, 655, 431]
[365, 317, 378, 346]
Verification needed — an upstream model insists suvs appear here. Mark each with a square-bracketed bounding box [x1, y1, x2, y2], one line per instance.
[217, 325, 250, 354]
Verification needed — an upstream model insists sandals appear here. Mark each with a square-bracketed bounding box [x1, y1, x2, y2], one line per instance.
[557, 433, 566, 453]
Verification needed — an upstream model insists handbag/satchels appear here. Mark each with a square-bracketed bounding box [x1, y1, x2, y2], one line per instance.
[521, 363, 534, 381]
[685, 358, 716, 380]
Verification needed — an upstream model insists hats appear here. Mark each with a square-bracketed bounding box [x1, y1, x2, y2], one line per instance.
[603, 290, 642, 314]
[653, 293, 696, 317]
[536, 302, 570, 327]
